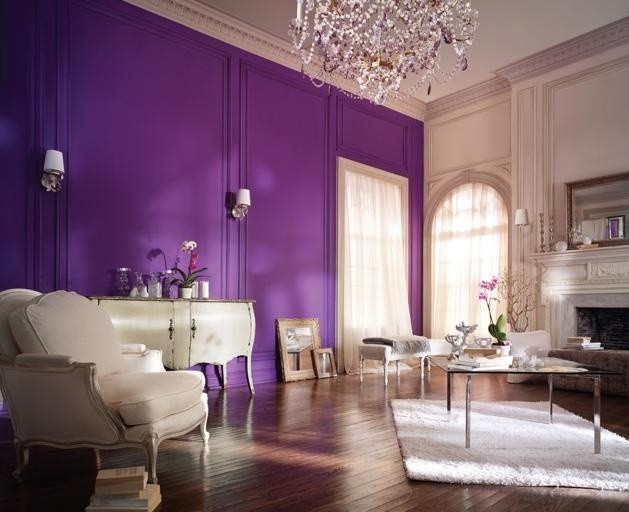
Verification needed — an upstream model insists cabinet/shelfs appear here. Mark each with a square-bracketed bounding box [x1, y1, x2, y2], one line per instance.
[89, 296, 257, 395]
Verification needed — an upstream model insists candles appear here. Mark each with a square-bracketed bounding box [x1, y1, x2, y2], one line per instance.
[548, 183, 554, 216]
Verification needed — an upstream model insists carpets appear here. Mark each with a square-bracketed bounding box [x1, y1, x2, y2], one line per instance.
[388, 398, 629, 491]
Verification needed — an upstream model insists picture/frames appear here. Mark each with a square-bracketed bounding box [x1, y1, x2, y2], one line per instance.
[312, 347, 338, 379]
[275, 317, 322, 384]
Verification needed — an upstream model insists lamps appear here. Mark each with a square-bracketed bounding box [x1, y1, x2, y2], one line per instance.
[515, 208, 532, 233]
[41, 150, 65, 192]
[231, 188, 251, 221]
[288, 0, 481, 106]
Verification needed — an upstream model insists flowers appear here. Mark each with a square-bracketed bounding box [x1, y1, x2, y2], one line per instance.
[478, 274, 510, 346]
[166, 240, 211, 288]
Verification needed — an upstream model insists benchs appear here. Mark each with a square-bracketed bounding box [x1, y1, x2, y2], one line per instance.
[357, 337, 432, 387]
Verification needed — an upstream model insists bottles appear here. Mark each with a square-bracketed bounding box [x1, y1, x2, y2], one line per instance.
[556, 240, 568, 252]
[582, 236, 592, 245]
[115, 268, 133, 296]
[130, 271, 163, 298]
[200, 280, 209, 300]
[191, 280, 198, 299]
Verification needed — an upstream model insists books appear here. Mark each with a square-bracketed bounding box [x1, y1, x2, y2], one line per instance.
[560, 337, 604, 350]
[448, 348, 513, 371]
[85, 466, 162, 512]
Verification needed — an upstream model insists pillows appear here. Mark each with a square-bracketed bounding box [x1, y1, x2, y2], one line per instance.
[7, 291, 123, 375]
[507, 330, 551, 383]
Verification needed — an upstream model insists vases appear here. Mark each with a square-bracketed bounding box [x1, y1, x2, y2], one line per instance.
[491, 344, 510, 356]
[178, 287, 193, 299]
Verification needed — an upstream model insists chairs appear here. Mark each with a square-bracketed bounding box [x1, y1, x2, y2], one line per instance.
[0, 288, 210, 484]
